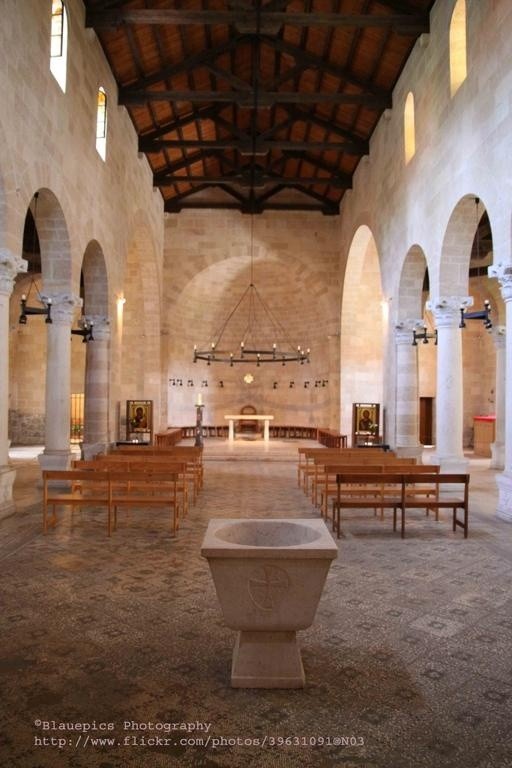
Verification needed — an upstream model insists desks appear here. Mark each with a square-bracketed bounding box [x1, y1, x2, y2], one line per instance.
[224, 414, 275, 451]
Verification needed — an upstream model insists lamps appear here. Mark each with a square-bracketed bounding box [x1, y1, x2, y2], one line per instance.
[410, 321, 437, 345]
[18, 292, 55, 326]
[458, 296, 494, 334]
[191, 213, 312, 368]
[69, 316, 96, 345]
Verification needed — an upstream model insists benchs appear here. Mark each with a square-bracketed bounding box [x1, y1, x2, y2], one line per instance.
[155, 423, 347, 448]
[42, 444, 203, 538]
[297, 446, 471, 539]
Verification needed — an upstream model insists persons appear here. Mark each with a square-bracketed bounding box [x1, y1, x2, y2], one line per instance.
[359, 410, 373, 431]
[132, 407, 147, 428]
